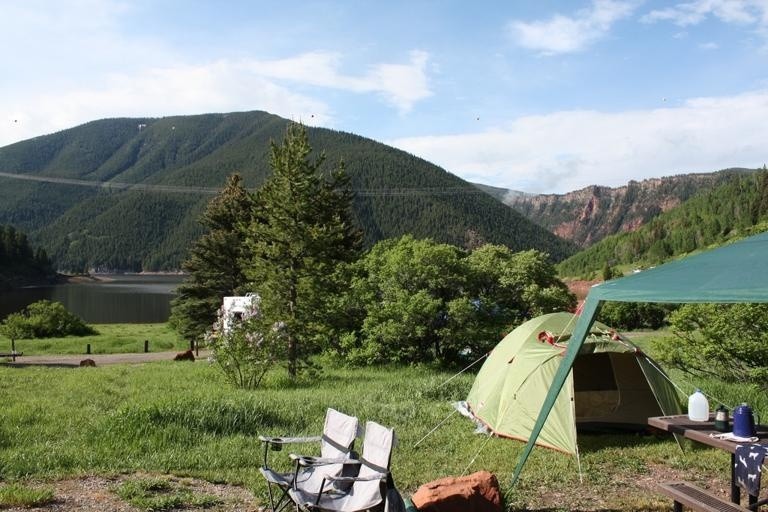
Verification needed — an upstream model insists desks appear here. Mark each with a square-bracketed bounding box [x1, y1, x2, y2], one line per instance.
[648, 410, 768, 512]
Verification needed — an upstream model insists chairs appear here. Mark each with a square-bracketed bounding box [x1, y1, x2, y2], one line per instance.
[259, 407, 395, 512]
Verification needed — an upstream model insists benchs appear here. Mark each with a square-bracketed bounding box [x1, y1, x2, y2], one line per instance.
[660, 481, 749, 512]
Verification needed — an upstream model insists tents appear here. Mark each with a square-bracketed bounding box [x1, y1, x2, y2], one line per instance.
[460, 307, 688, 456]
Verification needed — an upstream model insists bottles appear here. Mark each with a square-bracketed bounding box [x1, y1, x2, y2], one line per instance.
[715, 405, 729, 431]
[733, 405, 756, 437]
